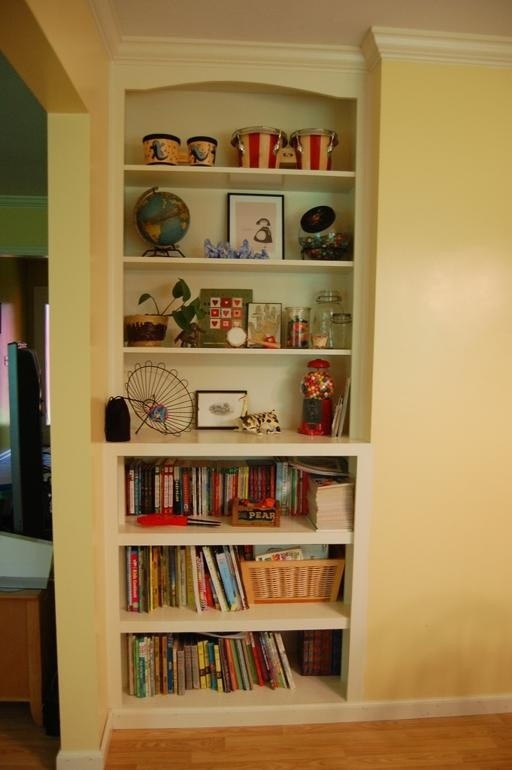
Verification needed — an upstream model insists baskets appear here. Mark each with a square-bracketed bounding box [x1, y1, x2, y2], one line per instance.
[239, 554, 345, 606]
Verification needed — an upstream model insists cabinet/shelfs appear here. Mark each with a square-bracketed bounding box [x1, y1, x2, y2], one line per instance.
[108, 57, 372, 440]
[102, 441, 375, 708]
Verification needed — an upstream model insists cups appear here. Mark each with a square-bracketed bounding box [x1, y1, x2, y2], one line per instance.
[143, 134, 219, 167]
[284, 306, 311, 350]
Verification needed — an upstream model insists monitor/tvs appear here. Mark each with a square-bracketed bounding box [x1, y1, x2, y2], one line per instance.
[5, 339, 51, 539]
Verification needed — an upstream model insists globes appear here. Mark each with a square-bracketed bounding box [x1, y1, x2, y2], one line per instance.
[133, 185, 190, 257]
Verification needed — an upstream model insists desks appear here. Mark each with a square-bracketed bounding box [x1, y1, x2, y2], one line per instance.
[1, 591, 45, 726]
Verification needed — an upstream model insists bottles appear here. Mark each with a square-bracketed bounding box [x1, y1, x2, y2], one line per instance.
[298, 206, 352, 261]
[297, 359, 336, 437]
[331, 312, 353, 349]
[310, 289, 344, 348]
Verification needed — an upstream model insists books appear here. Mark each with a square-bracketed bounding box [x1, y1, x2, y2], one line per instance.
[245, 545, 328, 598]
[125, 458, 353, 531]
[127, 631, 295, 698]
[298, 630, 341, 676]
[127, 546, 253, 613]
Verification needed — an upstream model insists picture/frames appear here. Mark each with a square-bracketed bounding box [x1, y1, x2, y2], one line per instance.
[195, 389, 247, 430]
[246, 302, 283, 348]
[227, 192, 285, 259]
